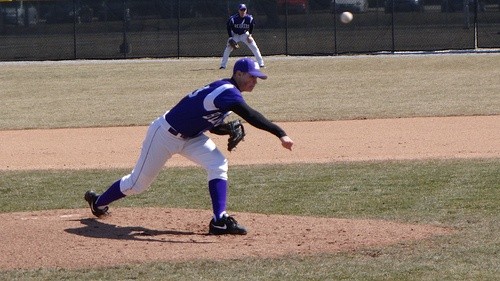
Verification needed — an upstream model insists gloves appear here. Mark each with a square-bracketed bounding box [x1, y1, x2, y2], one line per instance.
[247, 33, 253, 43]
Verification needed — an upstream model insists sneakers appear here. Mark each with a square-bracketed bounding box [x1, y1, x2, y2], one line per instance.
[84, 189, 110, 216]
[208, 215, 247, 235]
[219, 66, 226, 68]
[260, 65, 267, 68]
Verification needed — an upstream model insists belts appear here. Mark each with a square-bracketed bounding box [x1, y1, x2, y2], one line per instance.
[169, 127, 188, 140]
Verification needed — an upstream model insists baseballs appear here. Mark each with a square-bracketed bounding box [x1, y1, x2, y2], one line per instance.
[340, 11, 354, 23]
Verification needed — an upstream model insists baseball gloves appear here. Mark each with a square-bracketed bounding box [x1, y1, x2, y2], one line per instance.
[222, 120, 247, 150]
[229, 38, 239, 48]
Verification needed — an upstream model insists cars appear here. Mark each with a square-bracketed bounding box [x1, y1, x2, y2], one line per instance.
[47, 1, 93, 23]
[0, 0, 39, 25]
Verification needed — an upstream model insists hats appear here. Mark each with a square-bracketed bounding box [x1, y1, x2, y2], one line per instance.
[233, 56, 268, 79]
[239, 3, 247, 9]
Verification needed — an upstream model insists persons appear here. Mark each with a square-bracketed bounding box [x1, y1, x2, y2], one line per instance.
[219, 4, 268, 69]
[85, 56, 293, 235]
